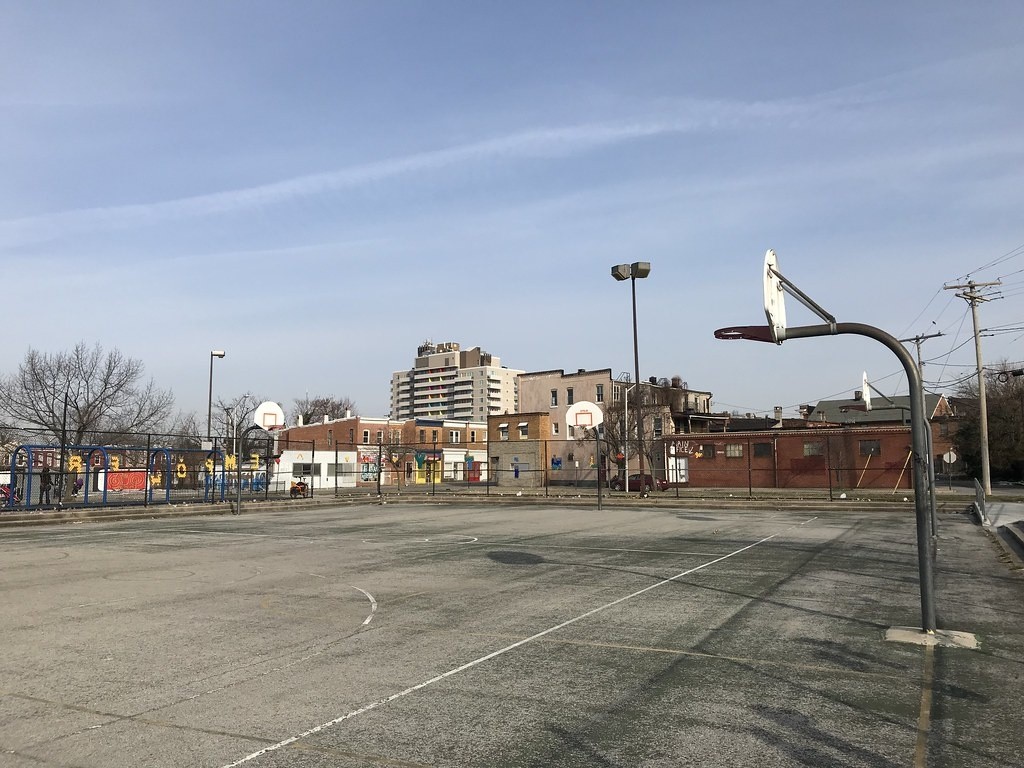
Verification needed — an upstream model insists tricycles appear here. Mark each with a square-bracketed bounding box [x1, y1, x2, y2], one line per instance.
[289, 475, 309, 499]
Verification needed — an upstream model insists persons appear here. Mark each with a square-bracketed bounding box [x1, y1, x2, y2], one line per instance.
[70, 478, 83, 497]
[39, 467, 53, 505]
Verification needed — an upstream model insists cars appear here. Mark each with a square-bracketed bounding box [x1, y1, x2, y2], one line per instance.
[609, 474, 671, 491]
